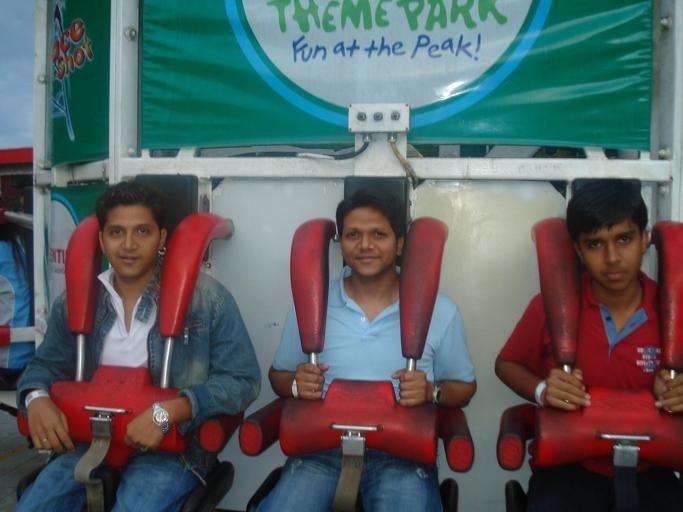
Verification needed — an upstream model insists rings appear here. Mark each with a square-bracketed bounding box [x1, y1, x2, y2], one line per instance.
[40, 438, 48, 443]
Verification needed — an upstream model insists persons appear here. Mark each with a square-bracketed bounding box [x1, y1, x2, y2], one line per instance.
[254, 187, 477, 512]
[494, 178, 683, 512]
[14, 182, 261, 512]
[0, 221, 34, 390]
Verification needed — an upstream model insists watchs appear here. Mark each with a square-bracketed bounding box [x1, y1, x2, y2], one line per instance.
[432, 382, 441, 405]
[151, 401, 170, 434]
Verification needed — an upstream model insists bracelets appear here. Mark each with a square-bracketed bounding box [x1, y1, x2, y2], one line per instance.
[292, 377, 298, 398]
